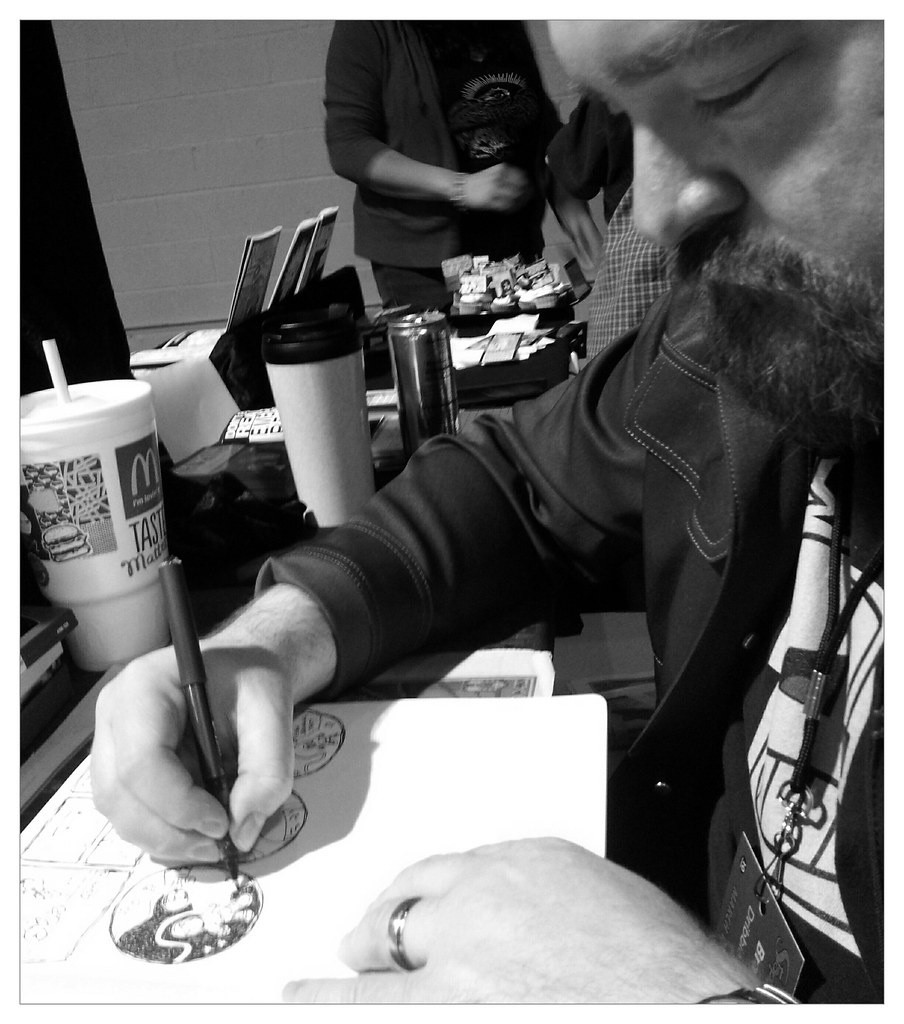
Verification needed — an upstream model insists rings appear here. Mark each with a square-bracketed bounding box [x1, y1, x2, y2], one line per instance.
[388, 897, 422, 971]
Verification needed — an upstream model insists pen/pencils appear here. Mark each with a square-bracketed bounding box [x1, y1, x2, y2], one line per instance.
[158, 558, 245, 895]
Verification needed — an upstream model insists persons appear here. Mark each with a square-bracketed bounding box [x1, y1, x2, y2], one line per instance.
[324, 20, 669, 361]
[94, 21, 885, 1005]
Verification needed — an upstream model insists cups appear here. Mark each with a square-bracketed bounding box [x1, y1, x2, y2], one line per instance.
[264, 315, 377, 530]
[19, 377, 170, 672]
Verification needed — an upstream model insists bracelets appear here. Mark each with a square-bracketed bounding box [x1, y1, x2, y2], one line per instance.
[697, 984, 799, 1004]
